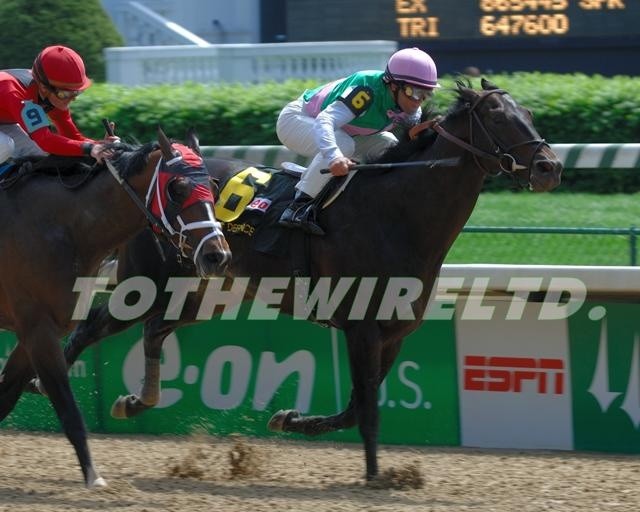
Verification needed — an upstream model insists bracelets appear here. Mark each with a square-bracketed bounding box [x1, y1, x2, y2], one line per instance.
[82, 142, 96, 154]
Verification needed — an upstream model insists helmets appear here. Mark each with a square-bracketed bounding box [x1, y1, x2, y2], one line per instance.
[29, 44, 95, 93]
[379, 46, 442, 91]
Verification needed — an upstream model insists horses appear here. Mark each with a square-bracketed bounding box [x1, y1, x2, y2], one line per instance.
[21, 70, 564, 483]
[0, 127, 234, 490]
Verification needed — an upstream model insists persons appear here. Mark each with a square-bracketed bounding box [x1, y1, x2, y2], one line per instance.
[0, 44, 122, 166]
[274, 46, 444, 236]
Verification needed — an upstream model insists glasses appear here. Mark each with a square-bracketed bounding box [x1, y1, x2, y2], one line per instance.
[396, 83, 435, 103]
[41, 81, 83, 101]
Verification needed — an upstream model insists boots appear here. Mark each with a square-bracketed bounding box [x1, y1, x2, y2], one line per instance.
[278, 192, 327, 237]
